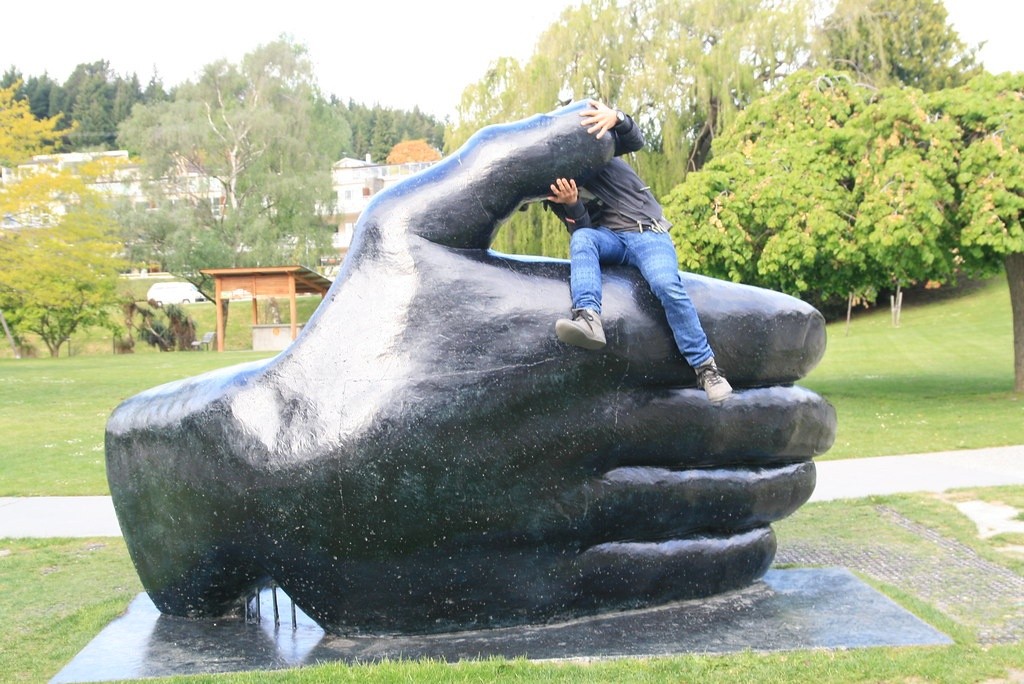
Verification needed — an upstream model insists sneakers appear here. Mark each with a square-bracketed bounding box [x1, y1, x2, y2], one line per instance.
[695, 358, 732, 402]
[555, 307, 606, 350]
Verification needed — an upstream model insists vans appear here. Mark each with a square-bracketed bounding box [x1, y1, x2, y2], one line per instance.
[146, 281, 199, 306]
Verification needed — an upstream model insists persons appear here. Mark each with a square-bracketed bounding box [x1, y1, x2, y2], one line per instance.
[104, 102, 839, 635]
[539, 99, 733, 402]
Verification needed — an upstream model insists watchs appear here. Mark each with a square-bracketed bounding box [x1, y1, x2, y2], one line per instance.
[616, 110, 625, 121]
